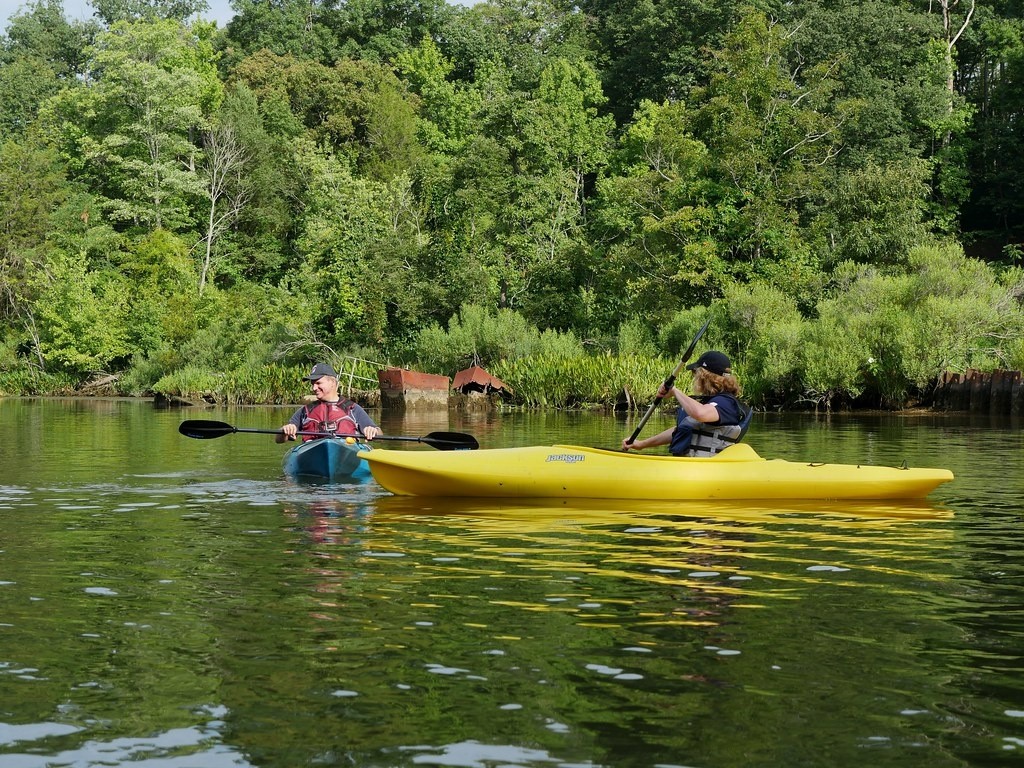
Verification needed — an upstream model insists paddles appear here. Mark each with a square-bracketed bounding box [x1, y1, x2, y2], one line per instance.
[178, 420, 479, 451]
[622, 318, 711, 453]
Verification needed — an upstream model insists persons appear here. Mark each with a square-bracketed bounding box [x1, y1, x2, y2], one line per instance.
[275, 363, 384, 443]
[622, 351, 753, 457]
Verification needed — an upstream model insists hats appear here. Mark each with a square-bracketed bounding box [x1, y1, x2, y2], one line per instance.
[686, 350, 731, 377]
[302, 363, 337, 382]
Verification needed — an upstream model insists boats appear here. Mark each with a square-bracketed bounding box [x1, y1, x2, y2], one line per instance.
[280, 440, 377, 486]
[356, 443, 958, 501]
